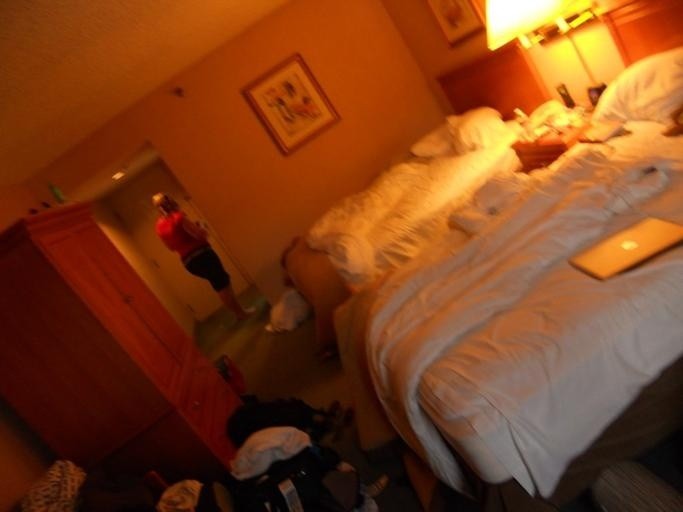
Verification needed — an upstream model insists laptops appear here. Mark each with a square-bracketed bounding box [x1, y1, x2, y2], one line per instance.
[567, 214, 683, 282]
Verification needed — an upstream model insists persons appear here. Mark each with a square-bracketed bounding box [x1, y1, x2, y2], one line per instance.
[150, 193, 256, 324]
